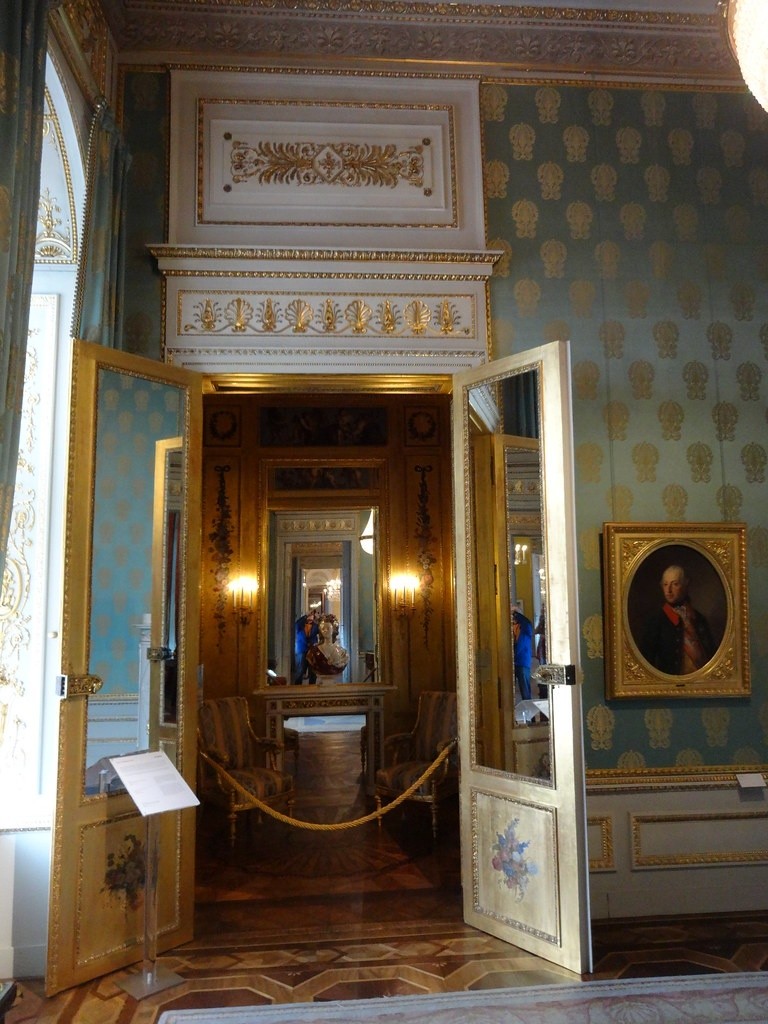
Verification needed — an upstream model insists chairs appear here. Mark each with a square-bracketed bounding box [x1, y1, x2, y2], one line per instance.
[372, 689, 457, 840]
[196, 695, 293, 843]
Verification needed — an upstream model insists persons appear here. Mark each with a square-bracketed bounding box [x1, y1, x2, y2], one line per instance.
[534, 601, 546, 666]
[307, 613, 320, 644]
[306, 614, 351, 685]
[293, 615, 311, 682]
[511, 606, 535, 701]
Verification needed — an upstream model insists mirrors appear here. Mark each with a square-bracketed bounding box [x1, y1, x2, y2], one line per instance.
[461, 361, 559, 791]
[268, 506, 381, 687]
[80, 360, 188, 803]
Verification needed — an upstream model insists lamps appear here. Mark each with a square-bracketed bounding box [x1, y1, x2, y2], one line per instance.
[723, 2, 768, 115]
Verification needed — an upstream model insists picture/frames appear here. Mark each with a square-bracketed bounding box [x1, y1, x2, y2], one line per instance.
[602, 522, 753, 697]
